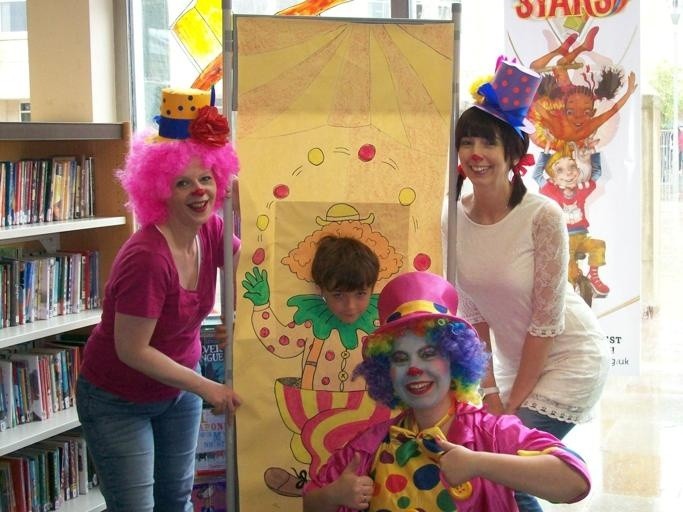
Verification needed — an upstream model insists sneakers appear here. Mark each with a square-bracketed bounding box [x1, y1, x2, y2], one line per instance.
[265, 468, 311, 497]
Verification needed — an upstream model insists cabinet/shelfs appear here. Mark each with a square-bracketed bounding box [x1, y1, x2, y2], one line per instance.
[0, 119, 131, 510]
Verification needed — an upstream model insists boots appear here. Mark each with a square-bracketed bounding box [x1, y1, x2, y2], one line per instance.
[587, 266, 610, 295]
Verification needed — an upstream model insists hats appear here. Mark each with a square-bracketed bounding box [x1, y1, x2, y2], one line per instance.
[146, 87, 212, 144]
[363, 272, 480, 358]
[473, 61, 541, 134]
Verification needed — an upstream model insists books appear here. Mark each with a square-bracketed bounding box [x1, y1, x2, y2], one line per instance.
[0, 155, 99, 512]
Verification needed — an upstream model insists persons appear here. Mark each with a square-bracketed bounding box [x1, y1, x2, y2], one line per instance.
[533, 139, 609, 307]
[302, 272, 592, 512]
[525, 27, 638, 141]
[311, 236, 380, 325]
[443, 104, 609, 512]
[75, 131, 242, 512]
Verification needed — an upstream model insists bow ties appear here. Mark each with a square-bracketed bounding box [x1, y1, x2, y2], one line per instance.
[389, 424, 447, 460]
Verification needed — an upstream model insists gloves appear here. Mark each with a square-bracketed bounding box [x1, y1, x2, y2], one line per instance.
[242, 266, 270, 305]
